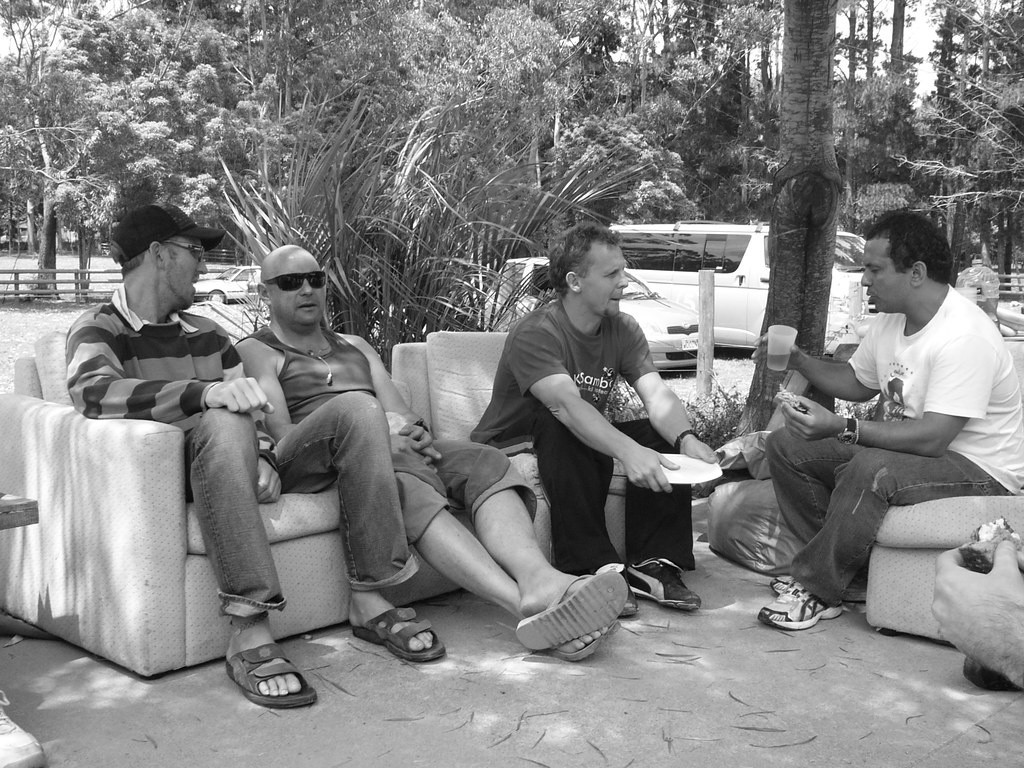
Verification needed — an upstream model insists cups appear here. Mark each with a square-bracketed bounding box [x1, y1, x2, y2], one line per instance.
[767, 324, 798, 372]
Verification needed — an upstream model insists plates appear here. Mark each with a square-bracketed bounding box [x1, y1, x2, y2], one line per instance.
[658, 452, 722, 483]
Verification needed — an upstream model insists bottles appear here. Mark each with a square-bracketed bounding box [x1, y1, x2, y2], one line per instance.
[957, 260, 998, 328]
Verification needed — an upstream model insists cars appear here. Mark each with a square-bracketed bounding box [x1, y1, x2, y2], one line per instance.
[193, 265, 262, 305]
[463, 257, 701, 371]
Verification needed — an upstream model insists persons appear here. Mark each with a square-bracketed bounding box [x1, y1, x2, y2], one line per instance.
[759, 210, 1023, 631]
[64, 204, 445, 710]
[230, 244, 629, 662]
[931, 540, 1024, 698]
[469, 221, 722, 617]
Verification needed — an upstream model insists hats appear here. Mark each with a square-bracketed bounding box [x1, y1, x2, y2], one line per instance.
[110, 203, 227, 266]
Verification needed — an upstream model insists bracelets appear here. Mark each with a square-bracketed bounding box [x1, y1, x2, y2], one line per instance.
[673, 429, 701, 454]
[412, 418, 430, 432]
[853, 419, 859, 444]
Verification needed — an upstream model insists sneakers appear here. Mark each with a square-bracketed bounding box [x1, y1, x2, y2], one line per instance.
[770, 576, 794, 594]
[594, 563, 639, 615]
[625, 557, 701, 610]
[757, 578, 843, 629]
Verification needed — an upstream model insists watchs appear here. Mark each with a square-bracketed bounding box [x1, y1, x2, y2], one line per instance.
[837, 418, 857, 446]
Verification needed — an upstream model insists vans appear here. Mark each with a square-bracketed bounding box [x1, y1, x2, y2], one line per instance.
[607, 220, 876, 346]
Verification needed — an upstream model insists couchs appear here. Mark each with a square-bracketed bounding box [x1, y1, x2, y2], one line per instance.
[1, 332, 465, 678]
[387, 331, 627, 569]
[865, 340, 1024, 646]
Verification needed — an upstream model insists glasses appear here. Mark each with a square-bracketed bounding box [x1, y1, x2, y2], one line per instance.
[164, 240, 204, 261]
[264, 271, 326, 291]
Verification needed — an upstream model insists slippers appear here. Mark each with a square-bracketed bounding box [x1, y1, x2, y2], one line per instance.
[516, 570, 628, 650]
[353, 607, 445, 662]
[543, 620, 621, 660]
[226, 644, 317, 708]
